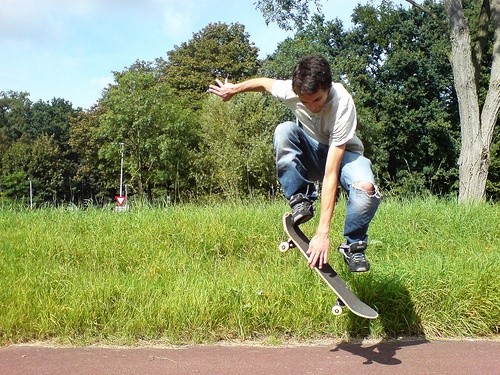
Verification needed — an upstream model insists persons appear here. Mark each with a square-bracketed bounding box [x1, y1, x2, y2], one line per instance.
[207, 54, 384, 273]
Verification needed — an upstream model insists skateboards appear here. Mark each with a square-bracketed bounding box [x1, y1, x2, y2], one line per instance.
[278, 212, 379, 320]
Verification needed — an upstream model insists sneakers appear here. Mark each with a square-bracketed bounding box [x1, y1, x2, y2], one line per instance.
[338, 241, 370, 272]
[288, 185, 318, 225]
